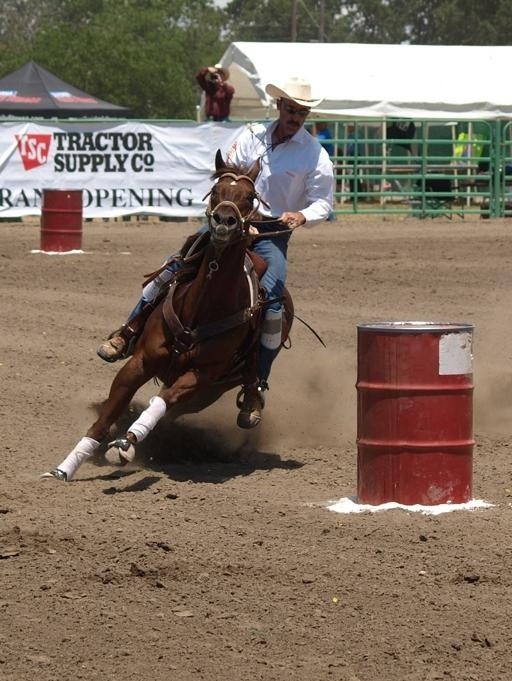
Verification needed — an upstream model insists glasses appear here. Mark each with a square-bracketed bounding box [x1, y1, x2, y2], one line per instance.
[282, 99, 310, 116]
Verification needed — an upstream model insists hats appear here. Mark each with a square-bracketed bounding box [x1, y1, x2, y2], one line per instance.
[265, 75, 325, 108]
[208, 64, 229, 82]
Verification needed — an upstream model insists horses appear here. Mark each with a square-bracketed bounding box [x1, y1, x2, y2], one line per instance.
[38, 147, 297, 483]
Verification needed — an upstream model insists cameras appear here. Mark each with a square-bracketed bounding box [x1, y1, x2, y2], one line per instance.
[210, 70, 219, 80]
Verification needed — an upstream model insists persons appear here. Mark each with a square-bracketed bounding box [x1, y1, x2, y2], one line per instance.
[307, 122, 364, 201]
[96, 75, 334, 428]
[194, 64, 235, 122]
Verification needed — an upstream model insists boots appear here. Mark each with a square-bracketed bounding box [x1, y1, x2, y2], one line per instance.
[237, 375, 265, 429]
[97, 322, 138, 362]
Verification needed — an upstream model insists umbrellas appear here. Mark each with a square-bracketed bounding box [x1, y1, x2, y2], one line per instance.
[0, 61, 128, 111]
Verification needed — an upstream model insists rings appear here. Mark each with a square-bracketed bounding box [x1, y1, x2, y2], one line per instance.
[288, 221, 294, 224]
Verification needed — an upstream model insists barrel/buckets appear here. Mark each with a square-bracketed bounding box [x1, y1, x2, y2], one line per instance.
[355, 321, 477, 505]
[39, 187, 83, 253]
